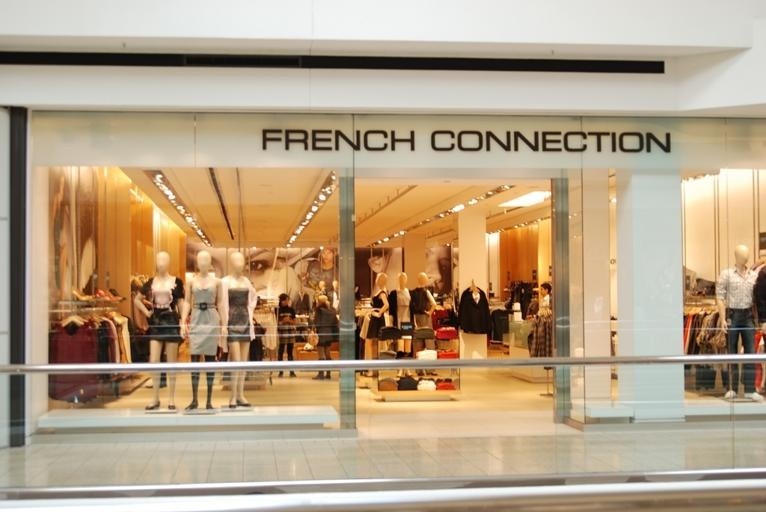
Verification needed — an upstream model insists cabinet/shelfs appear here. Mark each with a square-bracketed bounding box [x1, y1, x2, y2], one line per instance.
[373, 335, 461, 401]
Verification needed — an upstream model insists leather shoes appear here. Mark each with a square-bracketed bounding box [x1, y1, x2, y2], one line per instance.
[144, 400, 176, 410]
[229, 397, 252, 408]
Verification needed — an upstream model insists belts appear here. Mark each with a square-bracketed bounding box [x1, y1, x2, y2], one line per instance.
[192, 302, 217, 309]
[154, 303, 172, 309]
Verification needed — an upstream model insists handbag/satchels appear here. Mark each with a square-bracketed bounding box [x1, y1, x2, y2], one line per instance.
[307, 324, 320, 346]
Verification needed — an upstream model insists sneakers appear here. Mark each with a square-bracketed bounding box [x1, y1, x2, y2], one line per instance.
[278, 371, 330, 379]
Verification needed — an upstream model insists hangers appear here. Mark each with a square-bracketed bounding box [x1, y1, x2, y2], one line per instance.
[684, 301, 718, 316]
[537, 306, 552, 316]
[60, 303, 113, 327]
[469, 281, 479, 291]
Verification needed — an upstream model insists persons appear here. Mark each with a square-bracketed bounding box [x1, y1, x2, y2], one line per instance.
[311, 295, 339, 379]
[426, 247, 450, 294]
[386, 273, 412, 377]
[540, 284, 552, 311]
[134, 250, 184, 410]
[316, 247, 341, 281]
[318, 281, 328, 296]
[717, 244, 764, 401]
[368, 273, 389, 376]
[179, 250, 226, 409]
[193, 247, 318, 352]
[410, 273, 436, 377]
[328, 280, 339, 308]
[275, 293, 298, 377]
[218, 252, 257, 406]
